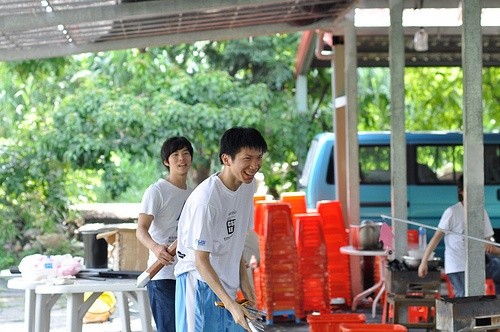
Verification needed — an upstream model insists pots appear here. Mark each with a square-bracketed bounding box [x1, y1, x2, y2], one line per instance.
[350, 219, 384, 251]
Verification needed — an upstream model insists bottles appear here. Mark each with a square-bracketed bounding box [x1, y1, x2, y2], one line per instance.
[419, 226, 427, 249]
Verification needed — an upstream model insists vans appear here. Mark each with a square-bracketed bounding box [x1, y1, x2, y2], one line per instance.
[295, 128, 499, 264]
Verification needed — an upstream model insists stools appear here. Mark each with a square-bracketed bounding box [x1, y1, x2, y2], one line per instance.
[254, 191, 351, 326]
[485, 278, 495, 296]
[374, 228, 455, 322]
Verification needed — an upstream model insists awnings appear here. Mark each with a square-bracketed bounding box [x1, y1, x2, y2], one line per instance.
[0, 0, 350, 60]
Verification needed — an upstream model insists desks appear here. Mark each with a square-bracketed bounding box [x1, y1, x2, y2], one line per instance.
[7, 275, 153, 332]
[341, 247, 393, 318]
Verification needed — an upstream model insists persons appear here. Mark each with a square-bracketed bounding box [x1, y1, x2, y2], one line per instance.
[175, 127, 267, 332]
[418, 174, 497, 299]
[136, 137, 193, 332]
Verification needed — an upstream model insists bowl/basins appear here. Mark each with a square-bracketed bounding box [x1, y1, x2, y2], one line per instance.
[407, 250, 436, 259]
[402, 255, 441, 270]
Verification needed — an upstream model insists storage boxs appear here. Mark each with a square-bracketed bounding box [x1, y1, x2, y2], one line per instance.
[97, 230, 148, 270]
[81, 223, 108, 269]
[339, 322, 408, 332]
[308, 313, 366, 332]
